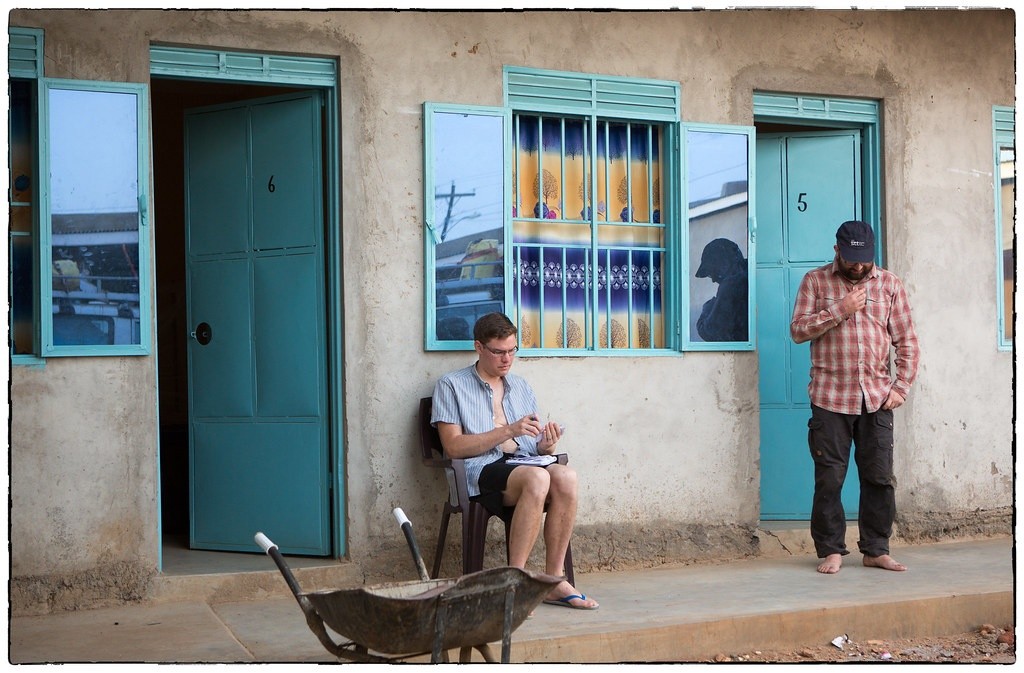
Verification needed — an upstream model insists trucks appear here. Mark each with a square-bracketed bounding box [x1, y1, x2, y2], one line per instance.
[51, 267, 141, 345]
[437, 260, 502, 341]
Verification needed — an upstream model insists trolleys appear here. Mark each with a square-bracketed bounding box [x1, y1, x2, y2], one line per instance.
[255, 507, 569, 665]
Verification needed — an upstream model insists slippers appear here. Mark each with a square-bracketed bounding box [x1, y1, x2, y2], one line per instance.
[544, 592, 600, 609]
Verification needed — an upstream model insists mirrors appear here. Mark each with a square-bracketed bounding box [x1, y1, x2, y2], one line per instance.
[998, 142, 1014, 346]
[37, 77, 153, 359]
[679, 121, 757, 352]
[421, 100, 514, 350]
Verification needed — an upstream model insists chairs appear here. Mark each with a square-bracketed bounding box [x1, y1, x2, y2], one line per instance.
[418, 395, 576, 589]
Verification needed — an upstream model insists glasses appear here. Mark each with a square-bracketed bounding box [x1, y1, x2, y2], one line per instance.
[845, 260, 870, 266]
[477, 340, 517, 357]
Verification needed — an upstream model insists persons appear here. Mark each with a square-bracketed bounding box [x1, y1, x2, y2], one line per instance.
[790, 221, 920, 574]
[436, 318, 469, 340]
[696, 238, 748, 341]
[430, 312, 599, 610]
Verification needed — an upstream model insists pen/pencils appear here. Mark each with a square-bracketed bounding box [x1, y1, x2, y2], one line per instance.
[530, 415, 536, 422]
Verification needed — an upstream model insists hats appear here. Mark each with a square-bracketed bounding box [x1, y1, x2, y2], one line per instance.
[836, 221, 875, 261]
[695, 238, 738, 277]
